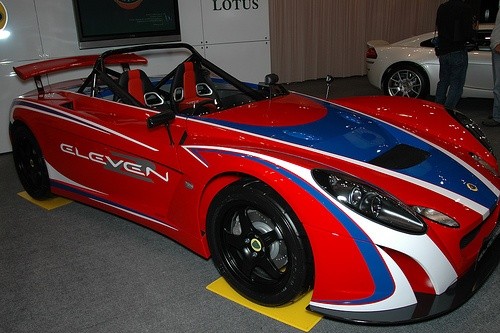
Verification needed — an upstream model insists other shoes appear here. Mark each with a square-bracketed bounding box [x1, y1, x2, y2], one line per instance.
[487, 108, 495, 115]
[483, 118, 499, 126]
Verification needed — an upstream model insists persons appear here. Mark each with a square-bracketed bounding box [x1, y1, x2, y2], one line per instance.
[433, 0, 480, 110]
[481, 8, 500, 126]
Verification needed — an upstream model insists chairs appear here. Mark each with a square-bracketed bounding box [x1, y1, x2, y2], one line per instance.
[113, 68, 167, 119]
[167, 61, 221, 117]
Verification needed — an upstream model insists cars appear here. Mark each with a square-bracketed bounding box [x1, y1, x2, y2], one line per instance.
[366, 22, 495, 100]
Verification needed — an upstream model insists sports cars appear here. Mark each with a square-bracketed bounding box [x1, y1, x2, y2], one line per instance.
[8, 41, 500, 326]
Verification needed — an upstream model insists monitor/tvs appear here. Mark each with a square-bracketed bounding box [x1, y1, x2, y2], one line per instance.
[72, 0, 181, 50]
